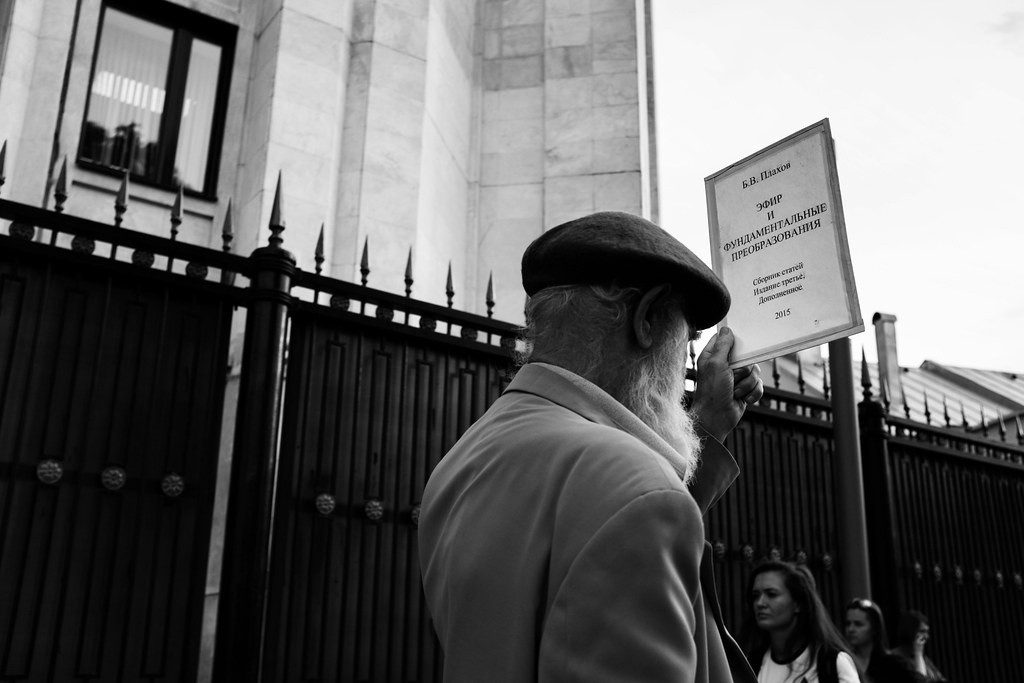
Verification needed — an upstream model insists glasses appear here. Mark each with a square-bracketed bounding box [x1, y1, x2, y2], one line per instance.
[852, 597, 871, 609]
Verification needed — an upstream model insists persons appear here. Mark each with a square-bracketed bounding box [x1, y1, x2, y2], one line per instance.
[889, 609, 948, 683]
[744, 558, 864, 683]
[844, 598, 914, 683]
[416, 212, 766, 683]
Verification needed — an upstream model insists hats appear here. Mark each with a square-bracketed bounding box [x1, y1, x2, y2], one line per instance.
[522, 211, 730, 331]
